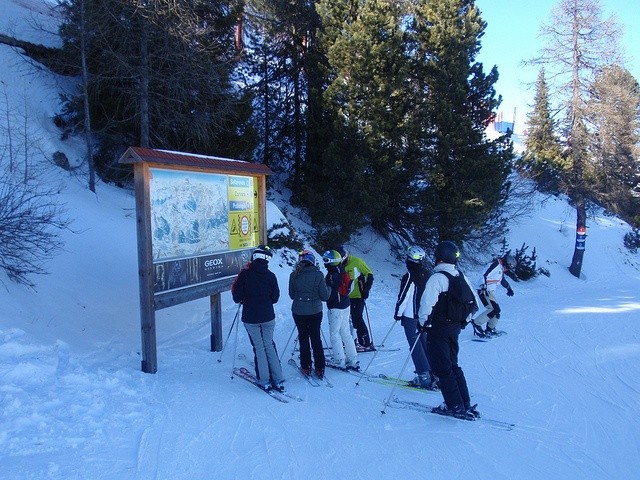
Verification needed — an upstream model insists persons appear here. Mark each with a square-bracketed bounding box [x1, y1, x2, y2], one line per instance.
[394, 244, 434, 386]
[471, 255, 517, 339]
[333, 245, 374, 351]
[415, 241, 479, 414]
[322, 250, 358, 367]
[288, 250, 333, 377]
[231, 245, 284, 391]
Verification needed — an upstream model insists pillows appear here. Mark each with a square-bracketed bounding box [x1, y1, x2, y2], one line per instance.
[406, 245, 427, 269]
[299, 249, 319, 267]
[503, 255, 517, 273]
[322, 250, 342, 267]
[335, 247, 349, 263]
[433, 241, 460, 263]
[250, 245, 273, 263]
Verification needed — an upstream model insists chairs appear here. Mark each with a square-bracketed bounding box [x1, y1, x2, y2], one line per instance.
[487, 328, 507, 336]
[327, 357, 346, 370]
[408, 372, 441, 391]
[470, 319, 484, 336]
[257, 378, 285, 394]
[353, 343, 371, 350]
[315, 365, 325, 379]
[437, 402, 471, 417]
[300, 363, 312, 377]
[347, 357, 360, 369]
